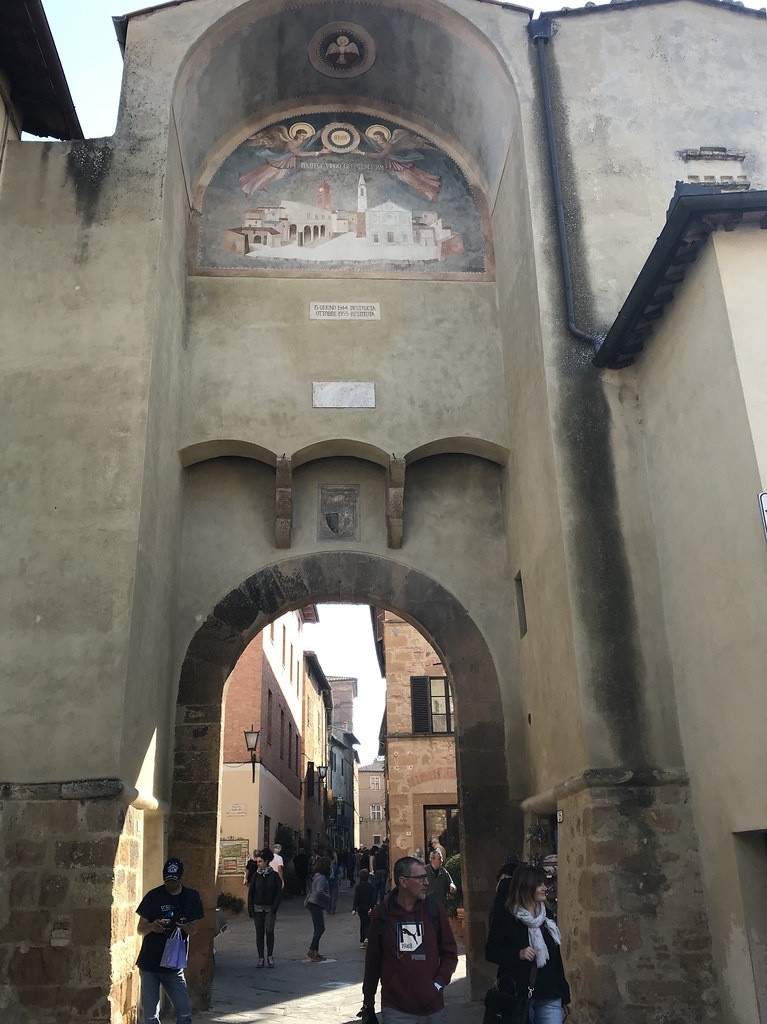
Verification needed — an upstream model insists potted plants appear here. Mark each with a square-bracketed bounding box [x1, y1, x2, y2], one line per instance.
[445, 854, 466, 956]
[217, 892, 245, 915]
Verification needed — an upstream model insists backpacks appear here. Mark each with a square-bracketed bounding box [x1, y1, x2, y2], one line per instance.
[329, 867, 335, 880]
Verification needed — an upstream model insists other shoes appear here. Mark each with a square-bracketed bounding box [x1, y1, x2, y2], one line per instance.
[308, 948, 327, 962]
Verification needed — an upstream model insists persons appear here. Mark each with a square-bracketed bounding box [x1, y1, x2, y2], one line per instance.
[303, 857, 331, 961]
[425, 838, 458, 911]
[352, 869, 378, 949]
[269, 844, 284, 889]
[483, 855, 571, 1024]
[357, 857, 459, 1024]
[293, 845, 393, 913]
[243, 850, 282, 969]
[136, 857, 205, 1024]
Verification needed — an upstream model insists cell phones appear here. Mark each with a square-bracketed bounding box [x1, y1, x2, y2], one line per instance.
[180, 918, 187, 924]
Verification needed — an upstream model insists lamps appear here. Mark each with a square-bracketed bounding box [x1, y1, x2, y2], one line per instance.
[243, 724, 260, 783]
[300, 766, 329, 796]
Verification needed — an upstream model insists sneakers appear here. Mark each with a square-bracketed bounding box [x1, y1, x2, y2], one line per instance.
[360, 937, 369, 949]
[267, 955, 274, 968]
[257, 957, 264, 967]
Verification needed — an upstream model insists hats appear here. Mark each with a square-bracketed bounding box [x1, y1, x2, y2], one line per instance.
[163, 858, 184, 881]
[359, 869, 368, 881]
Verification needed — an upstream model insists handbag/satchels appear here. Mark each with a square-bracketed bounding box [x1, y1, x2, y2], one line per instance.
[160, 927, 187, 970]
[360, 1003, 379, 1024]
[483, 987, 530, 1024]
[304, 892, 311, 908]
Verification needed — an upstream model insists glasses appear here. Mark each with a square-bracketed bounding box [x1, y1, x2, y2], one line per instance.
[402, 874, 429, 882]
